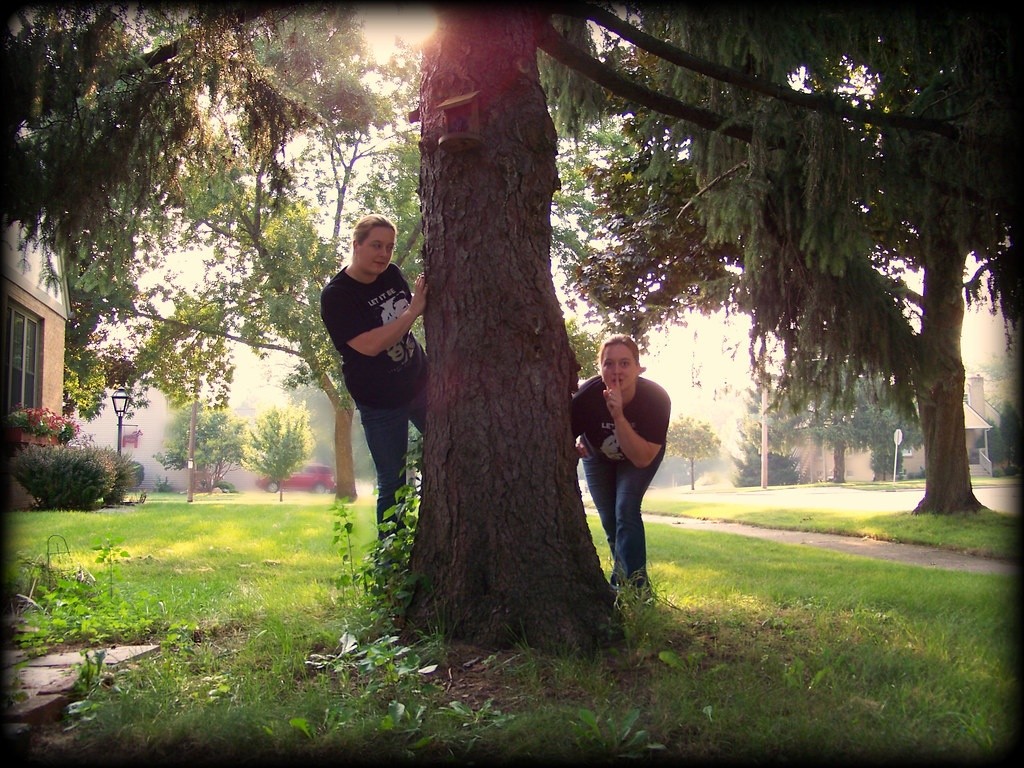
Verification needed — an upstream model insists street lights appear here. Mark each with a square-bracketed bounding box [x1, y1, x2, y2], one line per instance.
[110, 385, 133, 457]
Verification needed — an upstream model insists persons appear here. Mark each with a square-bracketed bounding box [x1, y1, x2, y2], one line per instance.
[320, 215, 429, 540]
[570, 333, 671, 594]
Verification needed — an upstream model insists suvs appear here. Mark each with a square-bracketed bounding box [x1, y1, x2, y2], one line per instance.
[254, 461, 337, 495]
